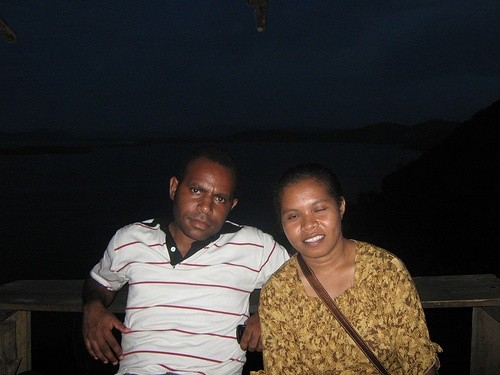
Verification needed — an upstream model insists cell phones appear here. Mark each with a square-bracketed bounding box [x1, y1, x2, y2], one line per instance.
[237, 325, 246, 344]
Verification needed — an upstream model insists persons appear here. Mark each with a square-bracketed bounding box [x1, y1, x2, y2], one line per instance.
[249, 165, 443, 375]
[82, 141, 287, 375]
